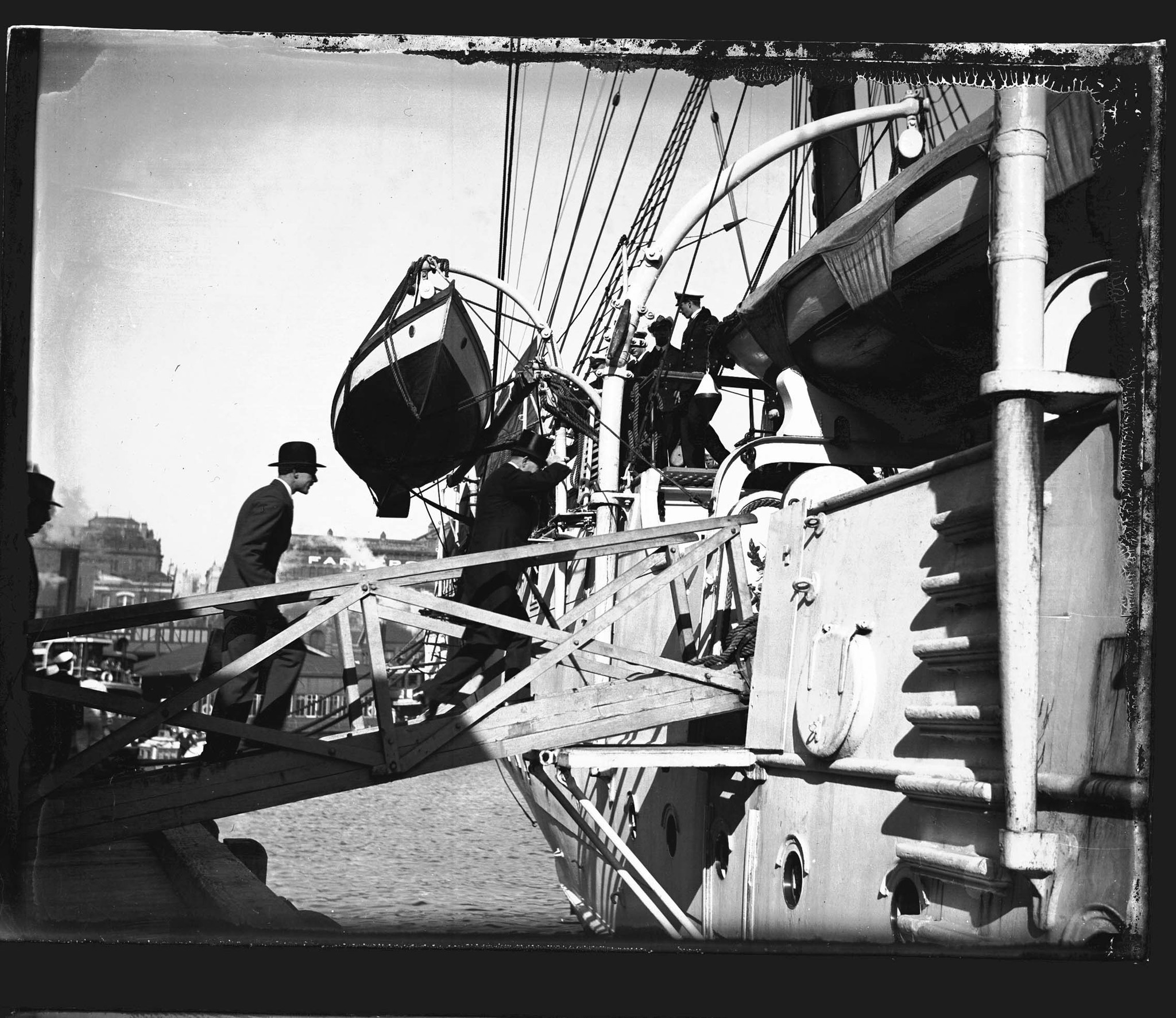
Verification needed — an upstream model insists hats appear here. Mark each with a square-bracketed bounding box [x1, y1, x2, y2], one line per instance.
[634, 331, 647, 339]
[674, 291, 704, 306]
[53, 651, 73, 666]
[505, 430, 554, 465]
[268, 442, 326, 468]
[648, 313, 675, 333]
[27, 472, 62, 507]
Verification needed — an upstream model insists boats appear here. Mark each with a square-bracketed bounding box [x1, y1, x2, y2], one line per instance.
[420, 52, 1148, 953]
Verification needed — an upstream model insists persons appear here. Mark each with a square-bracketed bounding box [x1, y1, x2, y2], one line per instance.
[197, 442, 325, 761]
[588, 354, 606, 422]
[115, 661, 121, 670]
[101, 657, 115, 668]
[675, 291, 735, 468]
[23, 472, 62, 670]
[87, 655, 95, 666]
[413, 430, 573, 720]
[618, 313, 680, 522]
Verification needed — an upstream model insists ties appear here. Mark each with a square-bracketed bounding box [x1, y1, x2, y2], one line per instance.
[659, 347, 662, 354]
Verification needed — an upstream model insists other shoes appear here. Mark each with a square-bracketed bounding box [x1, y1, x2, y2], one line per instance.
[413, 686, 438, 718]
